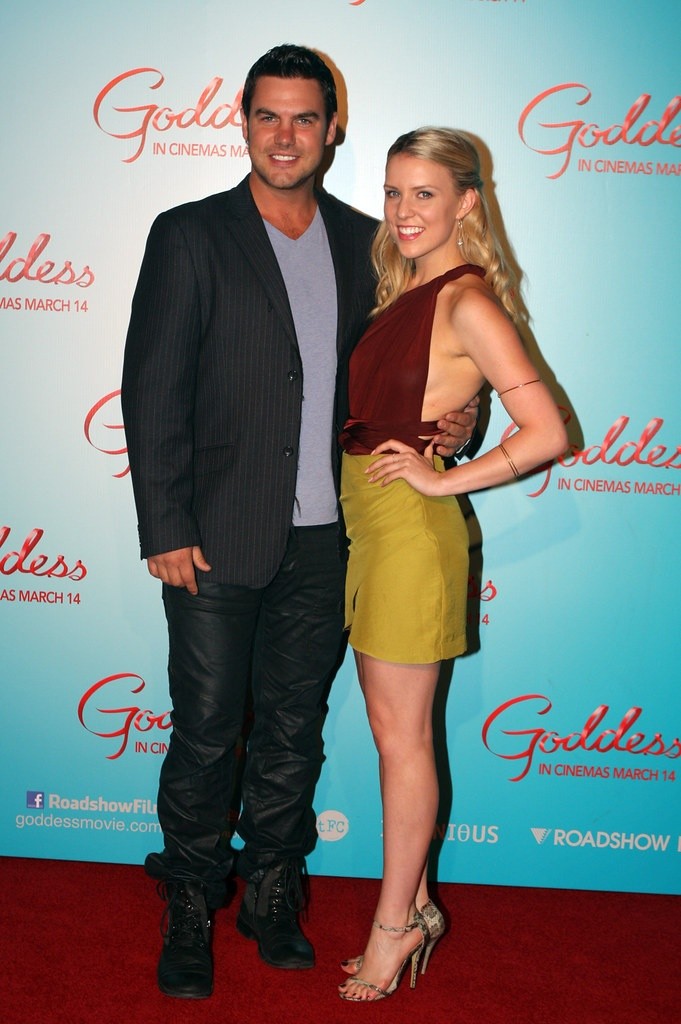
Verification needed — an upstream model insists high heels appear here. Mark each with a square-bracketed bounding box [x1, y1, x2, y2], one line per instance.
[338, 912, 430, 1001]
[340, 899, 445, 974]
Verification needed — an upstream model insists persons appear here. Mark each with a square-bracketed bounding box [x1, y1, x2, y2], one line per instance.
[122, 46, 481, 997]
[339, 126, 569, 1002]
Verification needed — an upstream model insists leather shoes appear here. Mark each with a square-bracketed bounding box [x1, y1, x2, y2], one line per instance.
[238, 865, 315, 968]
[157, 888, 213, 999]
